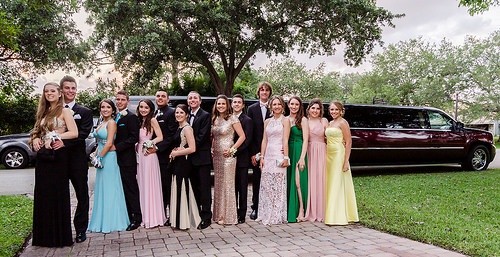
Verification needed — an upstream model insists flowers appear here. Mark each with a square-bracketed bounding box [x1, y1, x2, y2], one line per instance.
[144, 139, 154, 149]
[255, 152, 264, 162]
[30, 127, 62, 151]
[231, 148, 237, 157]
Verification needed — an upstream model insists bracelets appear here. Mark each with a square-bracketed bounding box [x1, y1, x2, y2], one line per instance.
[228, 147, 237, 155]
[52, 133, 60, 140]
[143, 141, 154, 148]
[284, 155, 290, 165]
[95, 155, 103, 161]
[260, 156, 264, 158]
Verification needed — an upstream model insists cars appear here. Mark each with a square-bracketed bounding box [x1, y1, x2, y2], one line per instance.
[285, 101, 496, 171]
[0, 116, 100, 169]
[97, 96, 260, 168]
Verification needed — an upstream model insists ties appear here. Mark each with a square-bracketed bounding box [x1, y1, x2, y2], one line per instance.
[155, 109, 161, 119]
[65, 105, 69, 108]
[115, 113, 121, 124]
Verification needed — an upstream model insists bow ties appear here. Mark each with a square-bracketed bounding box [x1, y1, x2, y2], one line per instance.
[260, 103, 268, 107]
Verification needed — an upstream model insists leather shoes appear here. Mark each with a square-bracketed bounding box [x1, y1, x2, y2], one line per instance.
[126, 222, 140, 231]
[164, 220, 171, 226]
[250, 211, 257, 220]
[197, 220, 211, 230]
[76, 233, 86, 243]
[238, 218, 245, 224]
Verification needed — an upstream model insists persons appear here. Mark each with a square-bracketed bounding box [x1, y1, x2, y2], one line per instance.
[302, 98, 328, 222]
[186, 91, 212, 230]
[134, 99, 168, 229]
[150, 89, 179, 227]
[30, 75, 93, 243]
[210, 95, 246, 226]
[247, 81, 275, 220]
[285, 96, 309, 223]
[231, 94, 254, 223]
[255, 95, 291, 226]
[109, 91, 142, 231]
[32, 83, 78, 248]
[88, 99, 130, 233]
[166, 104, 202, 231]
[324, 100, 359, 226]
